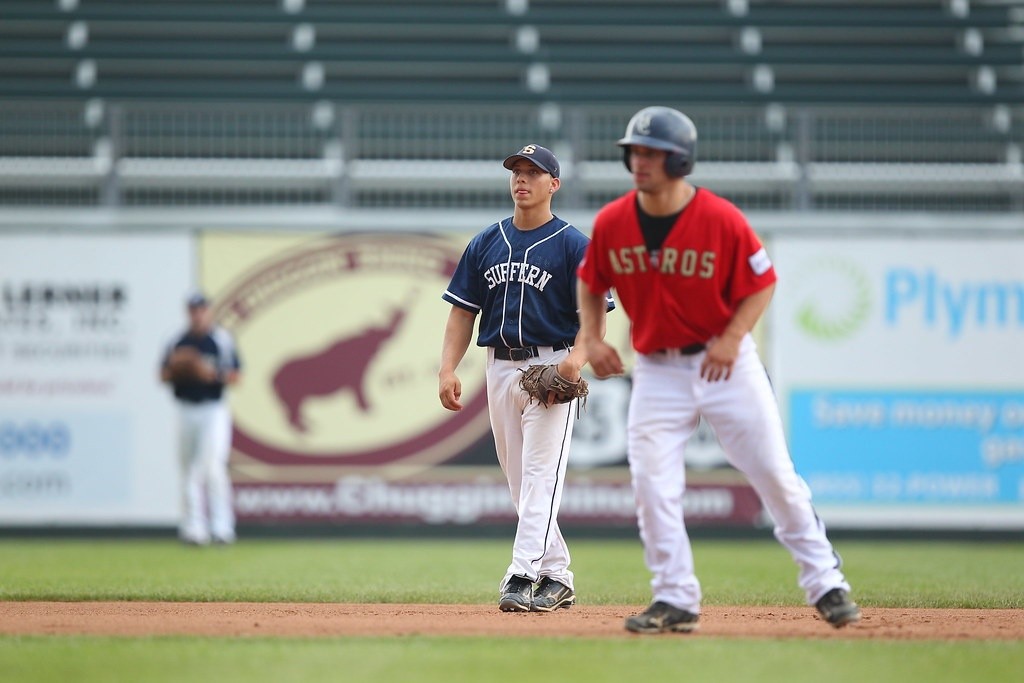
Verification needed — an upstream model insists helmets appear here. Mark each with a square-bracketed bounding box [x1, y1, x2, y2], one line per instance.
[615, 105, 699, 177]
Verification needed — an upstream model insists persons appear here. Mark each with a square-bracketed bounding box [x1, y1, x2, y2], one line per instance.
[575, 104, 859, 635]
[159, 296, 243, 547]
[437, 145, 616, 613]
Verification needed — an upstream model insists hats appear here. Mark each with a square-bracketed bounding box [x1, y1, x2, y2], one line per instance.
[502, 144, 559, 179]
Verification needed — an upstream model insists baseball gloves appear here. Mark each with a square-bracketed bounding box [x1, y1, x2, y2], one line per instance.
[519, 365, 589, 419]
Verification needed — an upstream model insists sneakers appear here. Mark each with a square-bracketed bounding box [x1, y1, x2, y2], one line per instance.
[623, 601, 701, 634]
[530, 577, 576, 611]
[815, 588, 863, 627]
[498, 574, 535, 611]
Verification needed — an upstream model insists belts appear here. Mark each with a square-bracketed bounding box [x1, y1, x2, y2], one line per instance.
[658, 342, 710, 360]
[493, 339, 574, 362]
